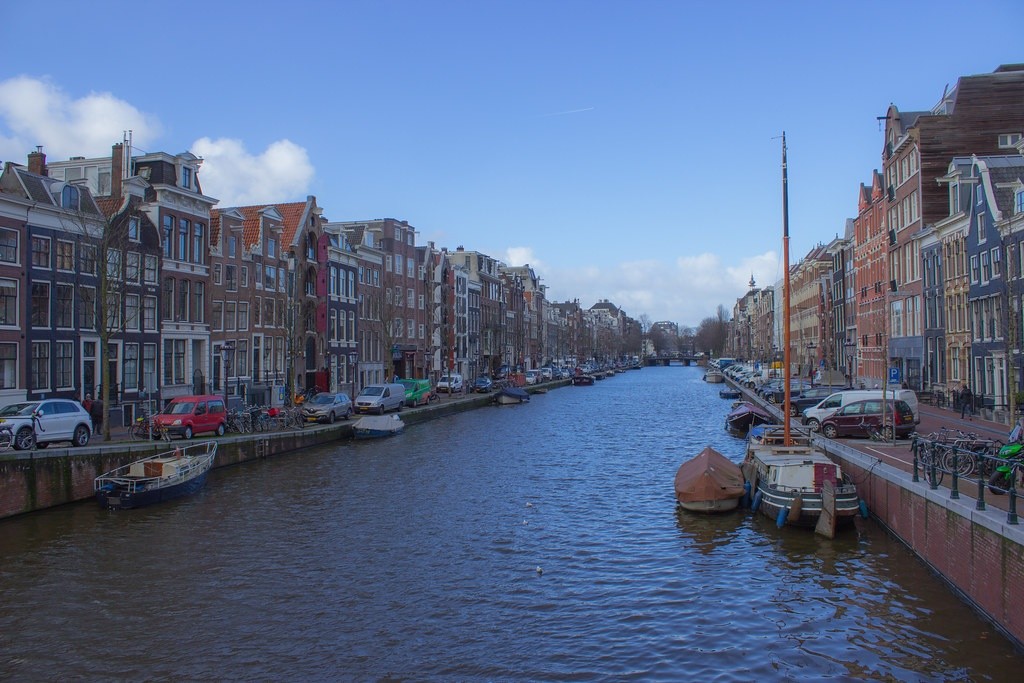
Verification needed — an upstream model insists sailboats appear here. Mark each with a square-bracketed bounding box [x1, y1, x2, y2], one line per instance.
[741, 130, 868, 536]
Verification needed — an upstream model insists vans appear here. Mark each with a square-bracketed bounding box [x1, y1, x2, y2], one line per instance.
[396, 379, 433, 408]
[144, 395, 228, 440]
[436, 374, 463, 393]
[820, 397, 915, 441]
[354, 383, 407, 413]
[801, 389, 921, 432]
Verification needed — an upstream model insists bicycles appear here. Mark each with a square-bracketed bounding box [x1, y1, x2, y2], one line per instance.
[910, 424, 1024, 499]
[226, 402, 305, 434]
[0, 423, 16, 453]
[127, 410, 171, 443]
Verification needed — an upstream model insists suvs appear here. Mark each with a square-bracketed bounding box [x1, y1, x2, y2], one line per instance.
[0, 398, 93, 451]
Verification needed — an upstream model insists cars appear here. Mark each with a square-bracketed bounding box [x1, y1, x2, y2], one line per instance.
[709, 357, 814, 405]
[299, 392, 353, 424]
[780, 386, 852, 417]
[468, 359, 639, 394]
[693, 352, 705, 357]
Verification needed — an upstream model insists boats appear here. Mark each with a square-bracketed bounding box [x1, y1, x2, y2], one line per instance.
[616, 368, 625, 373]
[705, 369, 723, 383]
[633, 365, 643, 369]
[592, 372, 606, 380]
[749, 420, 771, 445]
[606, 370, 615, 376]
[729, 397, 755, 410]
[724, 403, 774, 434]
[93, 441, 222, 511]
[493, 387, 531, 405]
[718, 387, 742, 401]
[572, 375, 595, 386]
[669, 445, 745, 514]
[350, 414, 406, 439]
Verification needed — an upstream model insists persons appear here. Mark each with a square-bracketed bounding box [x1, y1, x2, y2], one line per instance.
[814, 368, 817, 378]
[82, 392, 104, 435]
[959, 386, 973, 420]
[285, 384, 320, 406]
[384, 371, 399, 384]
[902, 381, 908, 389]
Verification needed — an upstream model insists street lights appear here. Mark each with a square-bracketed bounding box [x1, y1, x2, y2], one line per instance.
[550, 352, 554, 368]
[770, 342, 780, 378]
[804, 340, 816, 390]
[423, 348, 432, 379]
[506, 350, 513, 380]
[348, 348, 362, 415]
[759, 346, 765, 370]
[843, 337, 858, 391]
[218, 341, 237, 436]
[472, 350, 479, 396]
[751, 348, 756, 372]
